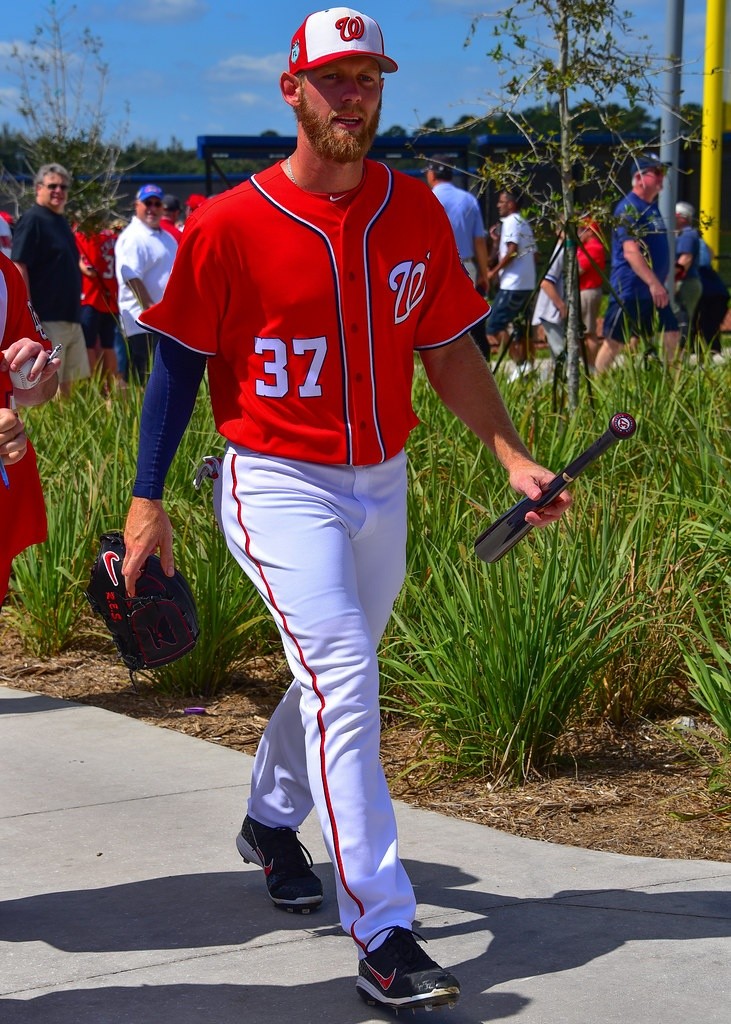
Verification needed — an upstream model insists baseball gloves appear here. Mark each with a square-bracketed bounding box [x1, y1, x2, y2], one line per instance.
[86, 529, 202, 675]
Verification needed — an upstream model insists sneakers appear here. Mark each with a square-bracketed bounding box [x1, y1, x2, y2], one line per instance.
[235, 814, 326, 916]
[354, 919, 463, 1016]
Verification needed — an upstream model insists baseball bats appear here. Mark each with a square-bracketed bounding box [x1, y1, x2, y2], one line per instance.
[475, 412, 638, 567]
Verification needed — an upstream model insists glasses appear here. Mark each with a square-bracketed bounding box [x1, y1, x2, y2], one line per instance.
[142, 198, 162, 206]
[41, 182, 69, 192]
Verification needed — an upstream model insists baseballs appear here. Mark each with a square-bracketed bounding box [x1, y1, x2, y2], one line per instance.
[10, 357, 43, 391]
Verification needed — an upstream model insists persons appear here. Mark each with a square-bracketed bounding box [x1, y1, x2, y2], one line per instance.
[10, 163, 92, 404]
[74, 217, 127, 398]
[675, 202, 731, 366]
[121, 7, 574, 1016]
[426, 156, 491, 293]
[0, 251, 60, 602]
[161, 195, 209, 241]
[487, 190, 539, 380]
[113, 184, 178, 384]
[530, 212, 605, 381]
[595, 157, 678, 377]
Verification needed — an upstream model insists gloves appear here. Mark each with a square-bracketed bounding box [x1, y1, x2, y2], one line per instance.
[191, 455, 224, 491]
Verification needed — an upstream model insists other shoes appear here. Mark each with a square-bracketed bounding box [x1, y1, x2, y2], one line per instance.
[711, 353, 727, 369]
[507, 361, 534, 383]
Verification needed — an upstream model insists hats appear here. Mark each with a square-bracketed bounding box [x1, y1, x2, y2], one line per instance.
[137, 184, 165, 200]
[184, 194, 206, 210]
[418, 152, 454, 174]
[627, 153, 672, 173]
[675, 202, 693, 221]
[287, 6, 399, 77]
[163, 194, 183, 214]
